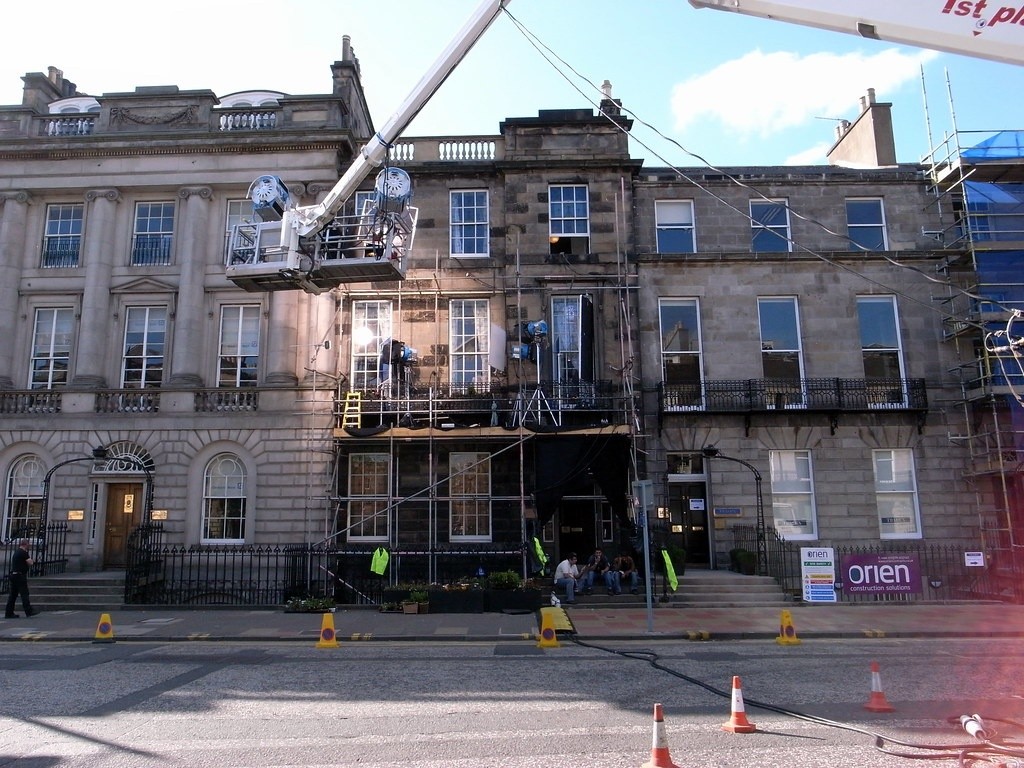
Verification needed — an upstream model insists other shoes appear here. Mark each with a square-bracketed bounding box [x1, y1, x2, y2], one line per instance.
[5, 615, 20, 618]
[587, 587, 592, 595]
[567, 599, 578, 604]
[632, 590, 638, 595]
[615, 591, 621, 595]
[26, 607, 39, 617]
[608, 588, 614, 596]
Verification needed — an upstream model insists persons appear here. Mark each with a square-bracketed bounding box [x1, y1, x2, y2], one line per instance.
[4, 538, 42, 619]
[586, 547, 616, 596]
[552, 551, 587, 605]
[612, 549, 639, 595]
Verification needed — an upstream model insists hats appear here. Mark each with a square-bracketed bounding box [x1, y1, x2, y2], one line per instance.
[566, 552, 576, 558]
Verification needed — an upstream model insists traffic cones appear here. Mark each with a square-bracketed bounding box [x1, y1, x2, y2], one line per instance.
[720, 675, 758, 734]
[90, 612, 117, 645]
[537, 611, 558, 649]
[315, 612, 341, 648]
[863, 663, 895, 711]
[644, 701, 680, 768]
[775, 609, 801, 646]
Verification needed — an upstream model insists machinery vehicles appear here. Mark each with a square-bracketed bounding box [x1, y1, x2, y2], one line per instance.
[219, 0, 1024, 297]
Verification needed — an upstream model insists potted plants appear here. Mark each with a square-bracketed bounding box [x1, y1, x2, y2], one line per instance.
[379, 569, 541, 616]
[284, 596, 337, 612]
[730, 548, 756, 575]
[669, 548, 687, 575]
